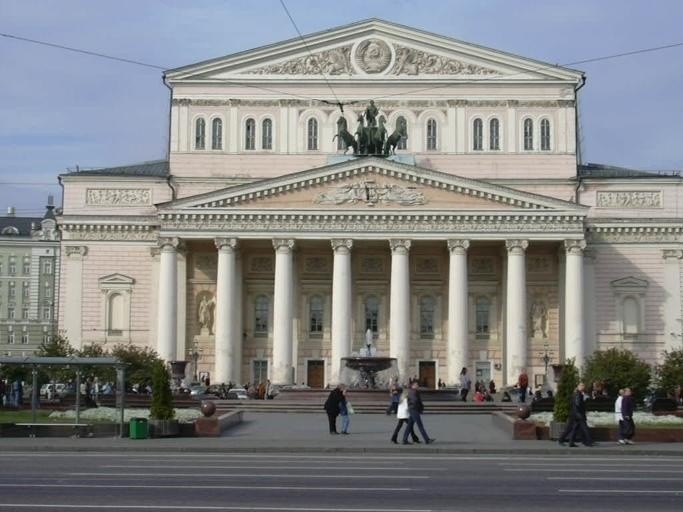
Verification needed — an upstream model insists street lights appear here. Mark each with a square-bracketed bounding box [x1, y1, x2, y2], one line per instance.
[186, 339, 204, 380]
[542, 342, 550, 375]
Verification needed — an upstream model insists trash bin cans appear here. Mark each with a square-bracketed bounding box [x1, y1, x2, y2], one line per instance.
[130, 417, 148, 439]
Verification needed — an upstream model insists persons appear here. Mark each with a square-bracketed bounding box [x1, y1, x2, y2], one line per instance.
[323, 384, 344, 435]
[403, 382, 434, 444]
[1, 372, 273, 410]
[390, 388, 420, 444]
[408, 366, 553, 402]
[197, 296, 213, 327]
[364, 97, 376, 126]
[338, 395, 354, 434]
[559, 387, 586, 447]
[570, 383, 595, 447]
[614, 390, 625, 446]
[620, 387, 634, 446]
[530, 299, 545, 330]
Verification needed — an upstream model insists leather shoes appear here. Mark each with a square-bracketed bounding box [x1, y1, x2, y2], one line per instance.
[557, 438, 637, 448]
[389, 436, 437, 446]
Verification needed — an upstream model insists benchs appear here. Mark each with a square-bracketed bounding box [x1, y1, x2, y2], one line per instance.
[14, 423, 94, 439]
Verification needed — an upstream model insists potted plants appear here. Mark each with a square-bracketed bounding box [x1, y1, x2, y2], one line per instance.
[548, 354, 580, 440]
[145, 356, 181, 437]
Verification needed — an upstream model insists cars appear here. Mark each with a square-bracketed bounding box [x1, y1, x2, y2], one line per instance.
[39, 382, 247, 399]
